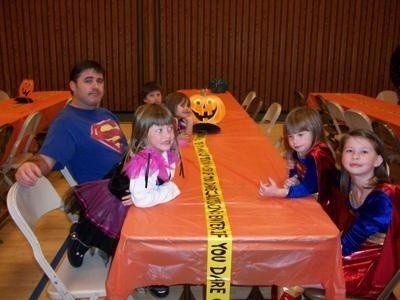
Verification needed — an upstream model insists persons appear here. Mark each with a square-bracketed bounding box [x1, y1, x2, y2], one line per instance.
[15, 60, 193, 268]
[271, 129, 400, 299]
[258, 106, 335, 216]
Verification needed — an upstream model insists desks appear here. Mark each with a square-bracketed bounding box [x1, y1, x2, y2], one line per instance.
[0, 91, 72, 230]
[116, 86, 342, 300]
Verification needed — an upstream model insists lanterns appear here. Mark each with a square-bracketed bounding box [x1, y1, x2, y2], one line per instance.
[189, 89, 225, 126]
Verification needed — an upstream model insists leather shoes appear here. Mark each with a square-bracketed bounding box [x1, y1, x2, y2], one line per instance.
[149, 286, 169, 297]
[67, 222, 92, 268]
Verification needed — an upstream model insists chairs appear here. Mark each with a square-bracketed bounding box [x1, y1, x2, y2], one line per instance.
[240, 89, 399, 300]
[1, 111, 136, 300]
